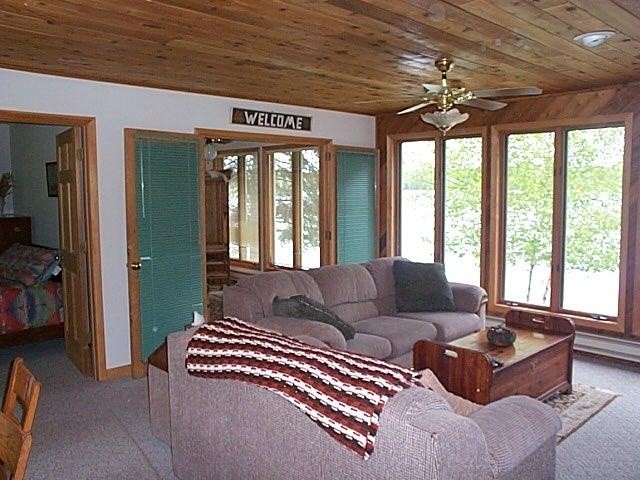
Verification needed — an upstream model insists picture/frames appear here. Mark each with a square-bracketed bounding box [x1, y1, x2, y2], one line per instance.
[45, 161, 59, 198]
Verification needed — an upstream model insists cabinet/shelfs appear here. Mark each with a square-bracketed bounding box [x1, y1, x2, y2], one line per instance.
[204, 167, 233, 294]
[0, 214, 33, 249]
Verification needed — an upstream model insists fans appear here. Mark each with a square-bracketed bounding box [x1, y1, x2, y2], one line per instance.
[348, 51, 545, 139]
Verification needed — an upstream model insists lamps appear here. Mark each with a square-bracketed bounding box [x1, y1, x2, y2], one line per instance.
[203, 137, 222, 162]
[573, 30, 616, 48]
[419, 58, 476, 137]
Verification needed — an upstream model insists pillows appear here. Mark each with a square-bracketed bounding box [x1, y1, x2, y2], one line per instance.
[0, 241, 63, 288]
[391, 259, 458, 314]
[269, 291, 358, 345]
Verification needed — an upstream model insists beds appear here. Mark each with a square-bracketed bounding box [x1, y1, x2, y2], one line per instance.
[0, 238, 64, 350]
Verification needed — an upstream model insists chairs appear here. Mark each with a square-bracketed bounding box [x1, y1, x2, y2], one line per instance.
[1, 353, 44, 480]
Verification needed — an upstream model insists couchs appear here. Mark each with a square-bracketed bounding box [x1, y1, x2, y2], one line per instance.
[146, 340, 172, 446]
[219, 256, 490, 372]
[165, 312, 564, 479]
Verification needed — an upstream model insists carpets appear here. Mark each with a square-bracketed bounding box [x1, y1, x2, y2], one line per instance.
[540, 384, 618, 448]
